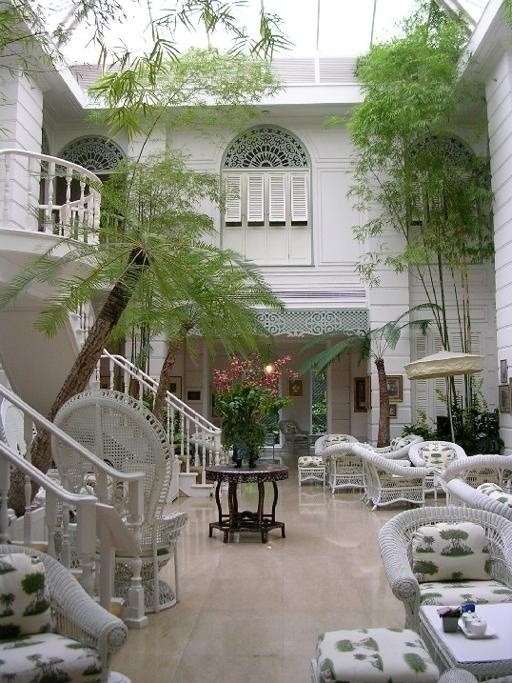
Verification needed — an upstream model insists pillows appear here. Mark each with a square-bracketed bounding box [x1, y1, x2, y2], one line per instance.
[1, 548, 53, 644]
[473, 478, 511, 506]
[406, 518, 493, 583]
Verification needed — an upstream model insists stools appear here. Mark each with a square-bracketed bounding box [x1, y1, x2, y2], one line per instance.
[297, 454, 327, 486]
[308, 623, 440, 682]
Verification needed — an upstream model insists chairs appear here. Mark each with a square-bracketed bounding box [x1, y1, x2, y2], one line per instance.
[47, 386, 189, 615]
[405, 439, 467, 497]
[444, 475, 512, 522]
[378, 502, 512, 634]
[0, 541, 128, 682]
[314, 432, 423, 495]
[440, 452, 512, 504]
[351, 445, 431, 512]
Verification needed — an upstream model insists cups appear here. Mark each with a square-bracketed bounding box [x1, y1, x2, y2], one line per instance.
[461, 610, 487, 634]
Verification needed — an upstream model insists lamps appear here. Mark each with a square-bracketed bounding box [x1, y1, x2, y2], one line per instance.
[264, 341, 274, 375]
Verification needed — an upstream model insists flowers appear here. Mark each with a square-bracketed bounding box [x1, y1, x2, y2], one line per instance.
[209, 348, 299, 459]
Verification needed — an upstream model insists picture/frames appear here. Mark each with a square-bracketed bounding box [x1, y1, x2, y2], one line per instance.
[288, 377, 305, 398]
[352, 373, 405, 419]
[184, 387, 204, 403]
[169, 374, 184, 398]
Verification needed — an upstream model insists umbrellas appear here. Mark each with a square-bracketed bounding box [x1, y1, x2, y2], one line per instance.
[403, 349, 485, 442]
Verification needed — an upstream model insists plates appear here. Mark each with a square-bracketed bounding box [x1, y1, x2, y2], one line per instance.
[456, 617, 496, 639]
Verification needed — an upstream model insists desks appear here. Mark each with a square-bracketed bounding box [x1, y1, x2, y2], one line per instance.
[431, 464, 443, 500]
[202, 460, 289, 543]
